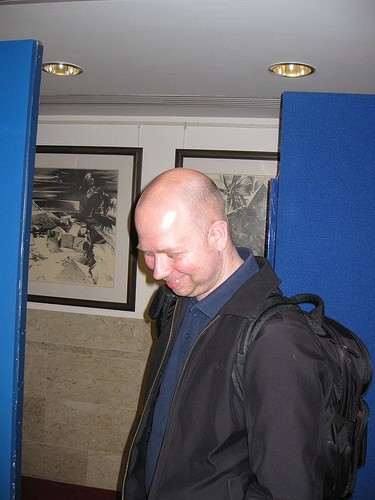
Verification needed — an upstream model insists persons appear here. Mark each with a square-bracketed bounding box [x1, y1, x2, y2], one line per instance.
[114, 167, 327, 500]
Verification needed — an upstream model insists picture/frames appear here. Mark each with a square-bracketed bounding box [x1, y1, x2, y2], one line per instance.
[25, 145, 143, 311]
[176, 148, 280, 260]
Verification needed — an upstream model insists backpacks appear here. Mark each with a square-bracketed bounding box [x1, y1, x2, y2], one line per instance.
[231, 293, 373, 500]
